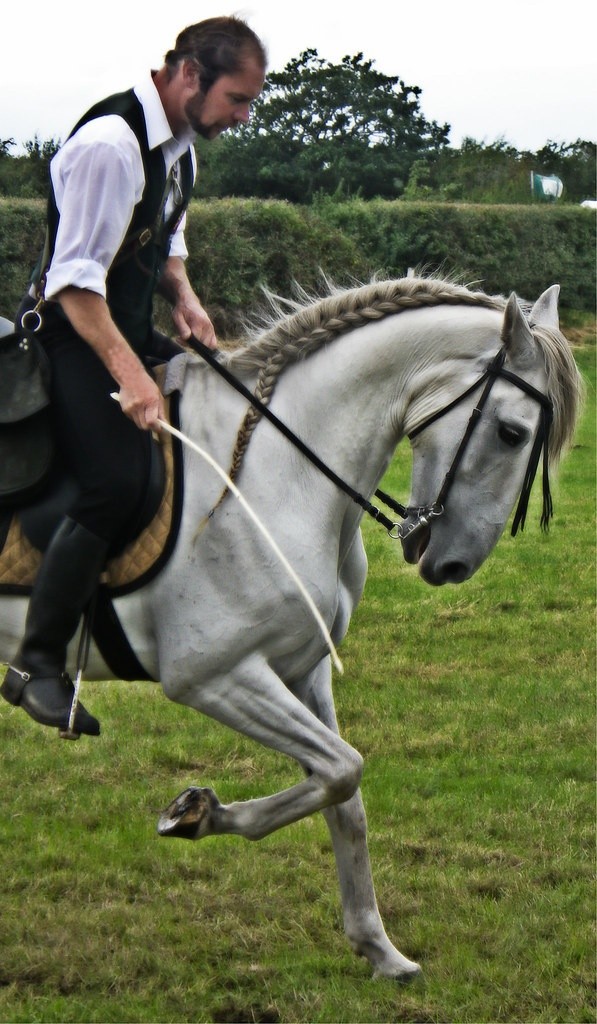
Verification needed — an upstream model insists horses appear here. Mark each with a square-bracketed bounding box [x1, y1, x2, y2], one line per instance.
[0, 256, 587, 978]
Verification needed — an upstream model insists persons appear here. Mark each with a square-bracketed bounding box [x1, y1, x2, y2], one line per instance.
[0, 17, 268, 737]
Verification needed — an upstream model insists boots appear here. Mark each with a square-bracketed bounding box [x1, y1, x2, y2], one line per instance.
[0, 513, 125, 736]
